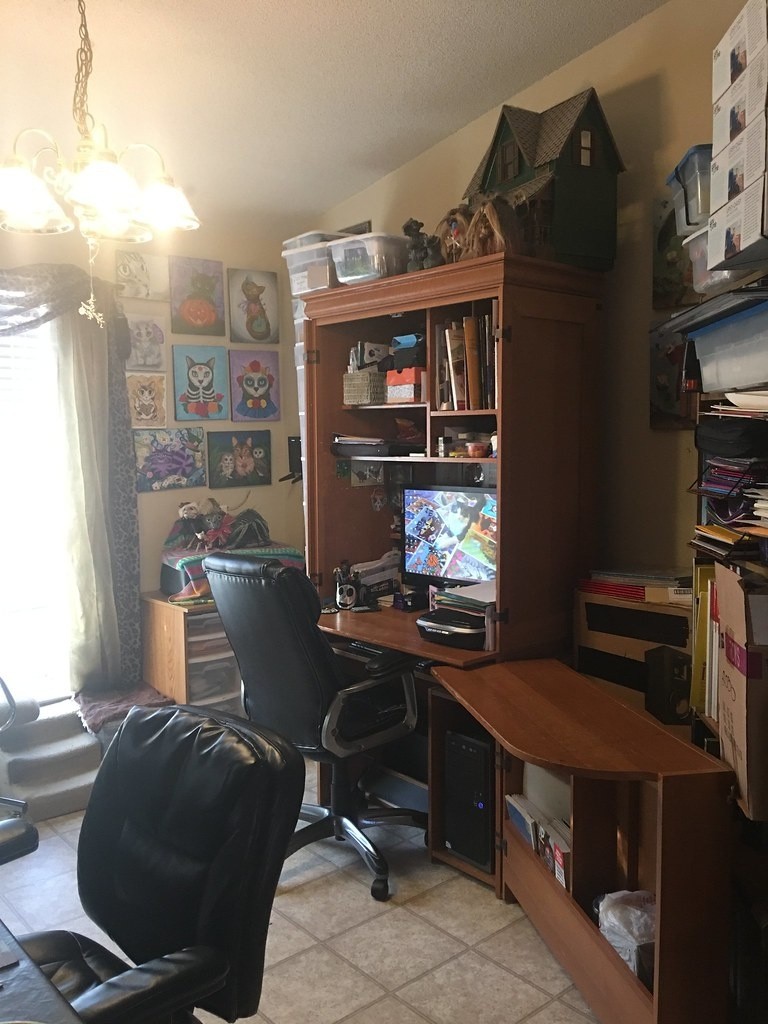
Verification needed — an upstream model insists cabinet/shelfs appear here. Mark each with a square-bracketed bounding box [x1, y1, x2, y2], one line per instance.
[434, 658, 735, 1024]
[570, 587, 696, 745]
[299, 253, 607, 901]
[145, 589, 246, 720]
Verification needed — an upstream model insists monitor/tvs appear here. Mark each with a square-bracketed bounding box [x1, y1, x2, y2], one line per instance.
[399, 484, 496, 587]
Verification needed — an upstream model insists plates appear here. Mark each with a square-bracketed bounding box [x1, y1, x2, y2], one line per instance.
[711, 406, 768, 412]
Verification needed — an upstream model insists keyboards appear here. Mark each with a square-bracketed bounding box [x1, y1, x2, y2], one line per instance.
[351, 640, 389, 653]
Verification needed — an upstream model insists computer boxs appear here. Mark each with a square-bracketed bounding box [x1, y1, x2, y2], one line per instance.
[444, 728, 496, 875]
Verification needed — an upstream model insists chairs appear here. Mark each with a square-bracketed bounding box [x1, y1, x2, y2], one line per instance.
[0, 706, 306, 1024]
[202, 552, 428, 902]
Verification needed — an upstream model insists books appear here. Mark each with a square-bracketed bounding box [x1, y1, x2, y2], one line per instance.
[504, 794, 573, 892]
[346, 340, 390, 370]
[699, 455, 768, 530]
[434, 300, 496, 411]
[187, 613, 242, 717]
[579, 566, 693, 604]
[693, 559, 747, 722]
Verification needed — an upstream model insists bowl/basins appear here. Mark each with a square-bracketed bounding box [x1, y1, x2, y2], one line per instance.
[725, 391, 768, 408]
[466, 443, 489, 458]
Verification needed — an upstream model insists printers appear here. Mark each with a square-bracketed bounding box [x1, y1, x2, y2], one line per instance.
[416, 609, 486, 651]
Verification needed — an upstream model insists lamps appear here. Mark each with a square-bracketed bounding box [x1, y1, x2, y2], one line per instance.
[0, 0, 202, 329]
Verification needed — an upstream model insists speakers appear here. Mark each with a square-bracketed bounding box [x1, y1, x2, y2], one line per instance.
[644, 644, 692, 726]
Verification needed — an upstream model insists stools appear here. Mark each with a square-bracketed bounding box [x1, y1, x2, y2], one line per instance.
[76, 678, 175, 763]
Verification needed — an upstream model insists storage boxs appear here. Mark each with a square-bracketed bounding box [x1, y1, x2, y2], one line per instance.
[687, 297, 768, 392]
[281, 231, 412, 297]
[665, 1, 767, 295]
[712, 561, 768, 822]
